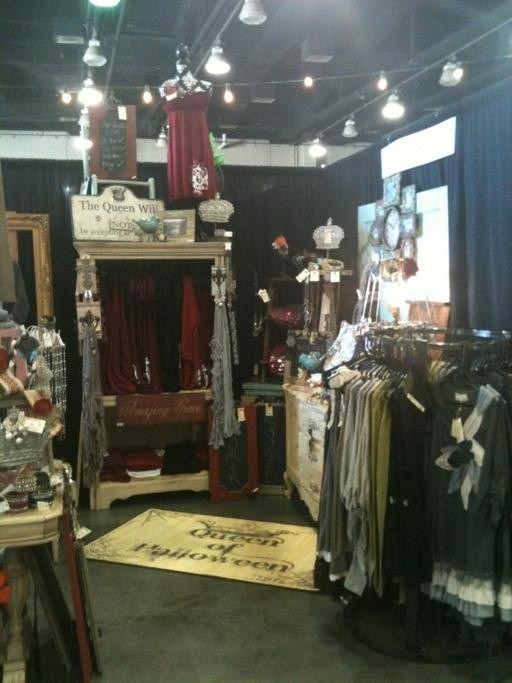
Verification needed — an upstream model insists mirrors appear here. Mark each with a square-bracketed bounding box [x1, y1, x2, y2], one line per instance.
[6, 211, 54, 330]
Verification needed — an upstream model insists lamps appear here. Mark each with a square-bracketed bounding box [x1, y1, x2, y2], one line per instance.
[77, 83, 103, 105]
[235, 1, 266, 25]
[380, 93, 406, 119]
[81, 39, 107, 67]
[204, 46, 231, 75]
[342, 118, 358, 136]
[76, 128, 94, 149]
[307, 140, 326, 156]
[439, 58, 464, 87]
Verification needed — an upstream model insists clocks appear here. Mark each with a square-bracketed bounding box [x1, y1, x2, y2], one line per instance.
[381, 207, 403, 248]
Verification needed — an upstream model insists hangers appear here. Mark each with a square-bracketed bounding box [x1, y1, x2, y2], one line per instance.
[358, 328, 510, 390]
